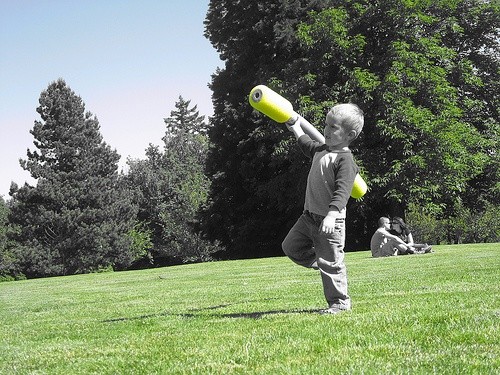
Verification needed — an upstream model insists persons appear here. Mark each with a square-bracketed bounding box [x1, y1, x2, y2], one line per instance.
[281, 103, 364, 314]
[369, 217, 432, 257]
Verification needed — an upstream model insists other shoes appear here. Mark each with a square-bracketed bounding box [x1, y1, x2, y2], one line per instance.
[425, 245, 432, 253]
[415, 250, 426, 254]
[319, 308, 341, 314]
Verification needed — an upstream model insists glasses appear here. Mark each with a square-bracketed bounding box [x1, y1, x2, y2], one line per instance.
[392, 222, 398, 224]
